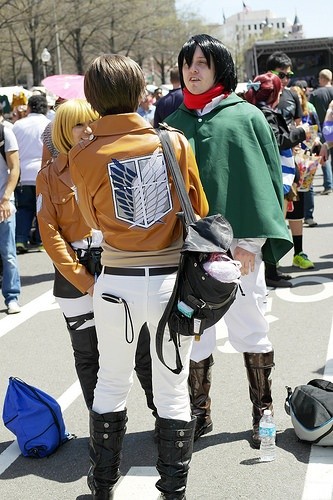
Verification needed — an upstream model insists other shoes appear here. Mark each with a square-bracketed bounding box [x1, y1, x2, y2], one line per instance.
[266, 273, 291, 286]
[7, 300, 20, 313]
[16, 243, 27, 253]
[322, 188, 332, 194]
[304, 217, 317, 226]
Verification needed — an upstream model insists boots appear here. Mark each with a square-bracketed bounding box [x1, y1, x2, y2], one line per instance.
[77, 406, 127, 500]
[152, 414, 197, 500]
[188, 353, 213, 441]
[243, 350, 277, 447]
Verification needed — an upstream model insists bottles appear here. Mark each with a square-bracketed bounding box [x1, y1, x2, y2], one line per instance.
[0, 203, 17, 223]
[259, 410, 277, 462]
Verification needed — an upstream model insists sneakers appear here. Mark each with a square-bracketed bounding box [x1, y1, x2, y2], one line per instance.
[295, 254, 315, 269]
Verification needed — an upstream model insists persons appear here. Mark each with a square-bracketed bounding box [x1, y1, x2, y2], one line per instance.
[245, 73, 311, 287]
[0, 69, 333, 314]
[267, 51, 316, 270]
[158, 34, 294, 449]
[37, 98, 161, 445]
[68, 53, 210, 500]
[0, 103, 22, 314]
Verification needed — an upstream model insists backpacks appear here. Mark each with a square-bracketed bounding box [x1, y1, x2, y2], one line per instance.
[285, 379, 333, 447]
[156, 128, 245, 375]
[3, 376, 66, 459]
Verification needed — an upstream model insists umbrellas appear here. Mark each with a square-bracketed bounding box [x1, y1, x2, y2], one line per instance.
[42, 75, 84, 99]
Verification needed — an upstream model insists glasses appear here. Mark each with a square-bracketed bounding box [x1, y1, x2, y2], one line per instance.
[270, 71, 295, 80]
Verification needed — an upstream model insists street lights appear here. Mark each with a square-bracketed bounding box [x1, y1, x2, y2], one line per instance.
[41, 47, 51, 77]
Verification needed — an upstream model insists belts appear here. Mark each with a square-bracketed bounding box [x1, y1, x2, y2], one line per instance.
[104, 266, 177, 276]
